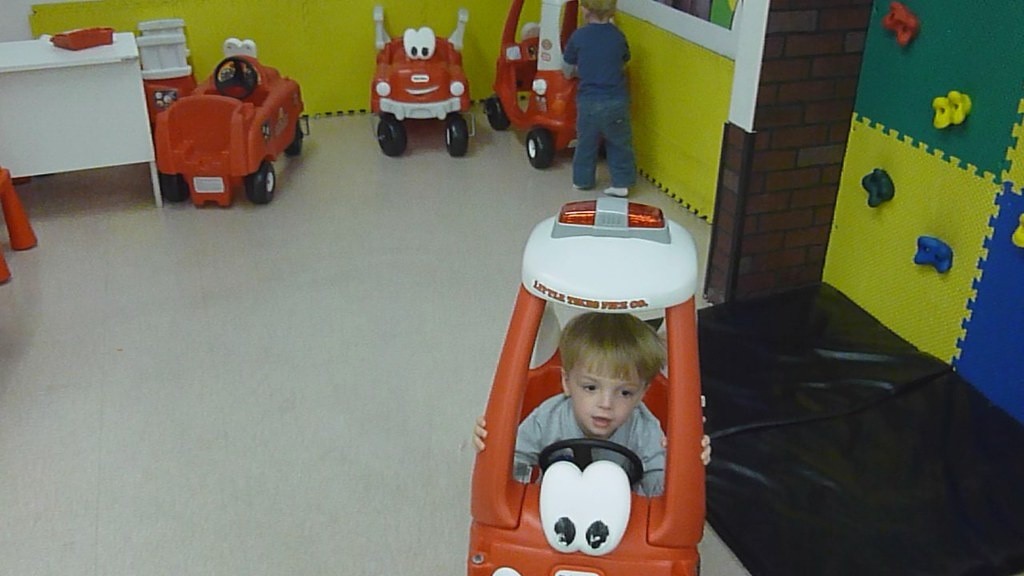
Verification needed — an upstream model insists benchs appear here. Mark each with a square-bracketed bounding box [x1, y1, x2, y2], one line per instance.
[695, 283, 1024, 576]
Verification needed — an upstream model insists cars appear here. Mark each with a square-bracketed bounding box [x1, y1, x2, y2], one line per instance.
[466, 198, 706, 576]
[155, 38, 311, 210]
[372, 5, 470, 157]
[481, 0, 607, 169]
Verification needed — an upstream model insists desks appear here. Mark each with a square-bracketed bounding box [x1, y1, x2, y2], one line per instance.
[0, 33, 164, 208]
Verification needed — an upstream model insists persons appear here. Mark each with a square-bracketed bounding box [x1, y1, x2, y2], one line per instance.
[562, 0, 636, 197]
[472, 313, 711, 497]
